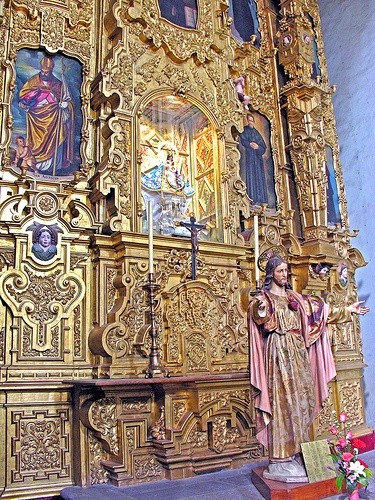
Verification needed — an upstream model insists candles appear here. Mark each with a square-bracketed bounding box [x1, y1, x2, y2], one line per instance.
[146, 199, 156, 285]
[253, 215, 262, 290]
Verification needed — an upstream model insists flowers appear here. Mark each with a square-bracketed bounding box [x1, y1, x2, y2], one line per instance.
[325, 412, 374, 500]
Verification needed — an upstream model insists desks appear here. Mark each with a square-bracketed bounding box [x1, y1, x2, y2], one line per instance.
[60, 368, 252, 398]
